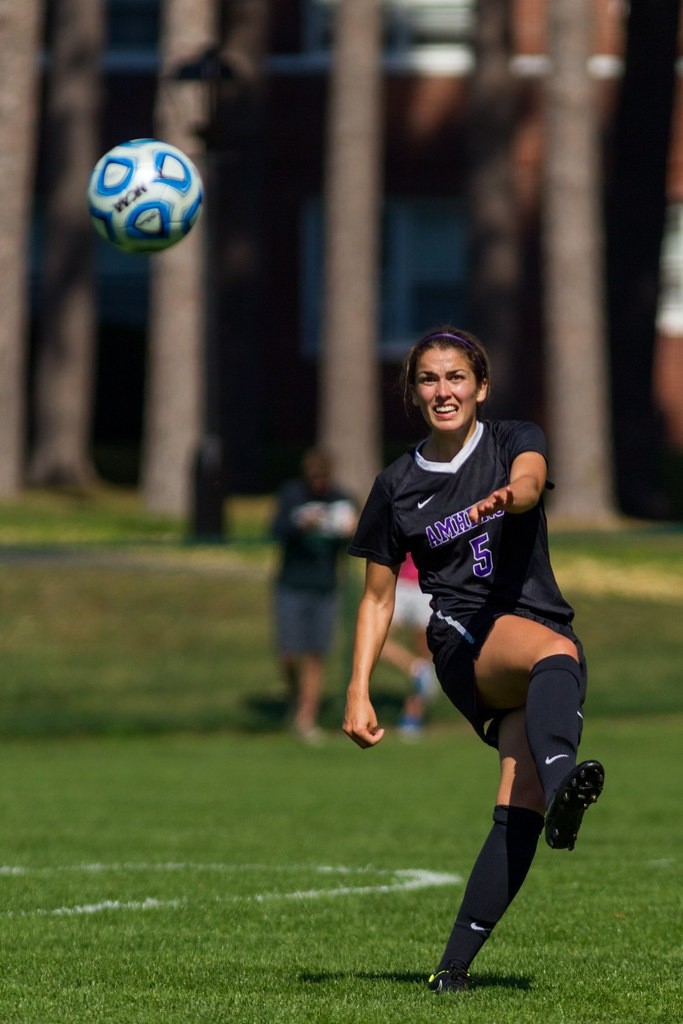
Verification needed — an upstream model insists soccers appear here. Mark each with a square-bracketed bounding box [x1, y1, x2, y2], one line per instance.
[88, 137, 204, 253]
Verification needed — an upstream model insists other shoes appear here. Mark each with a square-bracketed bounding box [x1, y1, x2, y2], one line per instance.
[396, 713, 426, 742]
[412, 661, 438, 698]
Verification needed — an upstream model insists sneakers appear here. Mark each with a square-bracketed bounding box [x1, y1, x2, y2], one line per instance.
[546, 760, 605, 851]
[427, 959, 481, 994]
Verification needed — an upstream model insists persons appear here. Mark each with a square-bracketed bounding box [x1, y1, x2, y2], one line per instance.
[269, 449, 361, 735]
[339, 326, 605, 991]
[377, 552, 437, 736]
